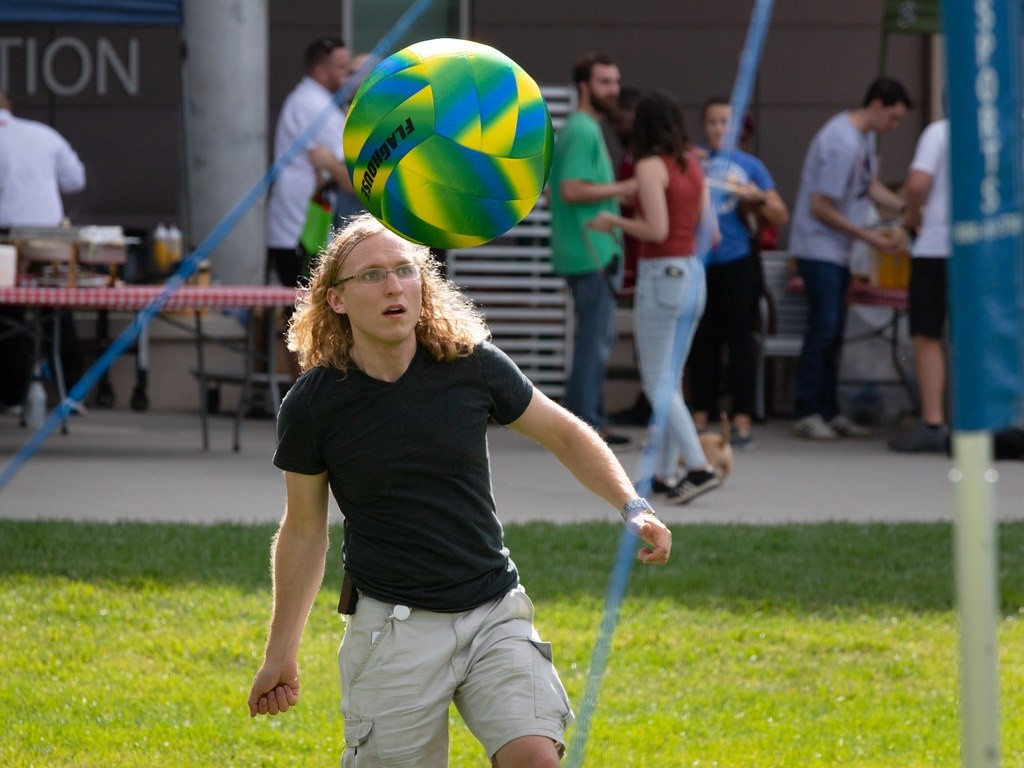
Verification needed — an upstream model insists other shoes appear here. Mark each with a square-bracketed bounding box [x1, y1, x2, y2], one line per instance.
[593, 428, 630, 448]
[792, 414, 839, 441]
[726, 426, 753, 449]
[828, 412, 875, 437]
[70, 400, 88, 416]
[887, 423, 949, 452]
[609, 403, 652, 426]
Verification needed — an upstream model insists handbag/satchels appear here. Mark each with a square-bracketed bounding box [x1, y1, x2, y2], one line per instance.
[299, 178, 338, 257]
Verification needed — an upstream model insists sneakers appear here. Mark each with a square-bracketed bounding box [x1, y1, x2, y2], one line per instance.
[636, 472, 684, 502]
[662, 465, 720, 504]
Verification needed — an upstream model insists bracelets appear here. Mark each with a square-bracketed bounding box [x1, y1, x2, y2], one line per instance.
[752, 191, 767, 210]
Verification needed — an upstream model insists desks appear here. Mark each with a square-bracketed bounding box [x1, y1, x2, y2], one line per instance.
[789, 275, 907, 425]
[0, 287, 311, 454]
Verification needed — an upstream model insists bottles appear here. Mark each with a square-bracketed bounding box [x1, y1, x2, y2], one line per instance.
[170, 225, 184, 264]
[28, 363, 46, 433]
[154, 222, 170, 273]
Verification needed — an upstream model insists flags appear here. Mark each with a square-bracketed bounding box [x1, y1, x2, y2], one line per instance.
[940, 0, 1024, 432]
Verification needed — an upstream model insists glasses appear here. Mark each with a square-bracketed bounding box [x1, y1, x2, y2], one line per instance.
[327, 261, 420, 289]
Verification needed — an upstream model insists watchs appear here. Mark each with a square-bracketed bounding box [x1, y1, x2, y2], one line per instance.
[621, 498, 656, 522]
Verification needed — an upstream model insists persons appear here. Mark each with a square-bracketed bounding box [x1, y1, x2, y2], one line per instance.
[0, 87, 85, 416]
[248, 215, 671, 768]
[788, 74, 952, 453]
[261, 36, 383, 417]
[548, 50, 787, 506]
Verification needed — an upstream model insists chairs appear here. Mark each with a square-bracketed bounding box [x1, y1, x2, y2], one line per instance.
[753, 252, 809, 423]
